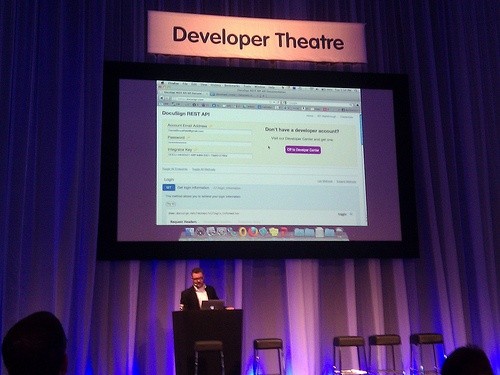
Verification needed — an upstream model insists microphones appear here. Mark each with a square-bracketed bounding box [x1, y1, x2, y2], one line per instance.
[195, 283, 197, 286]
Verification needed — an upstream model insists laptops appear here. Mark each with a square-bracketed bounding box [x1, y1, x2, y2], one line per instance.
[201, 299, 225, 311]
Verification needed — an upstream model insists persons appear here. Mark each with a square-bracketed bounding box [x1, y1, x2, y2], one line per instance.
[442, 345, 493, 375]
[180, 267, 235, 310]
[0, 311, 69, 375]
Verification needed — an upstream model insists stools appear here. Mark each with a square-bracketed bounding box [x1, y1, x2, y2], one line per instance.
[411, 333, 445, 374]
[368, 334, 401, 373]
[333, 335, 368, 373]
[195, 341, 225, 375]
[254, 338, 286, 375]
